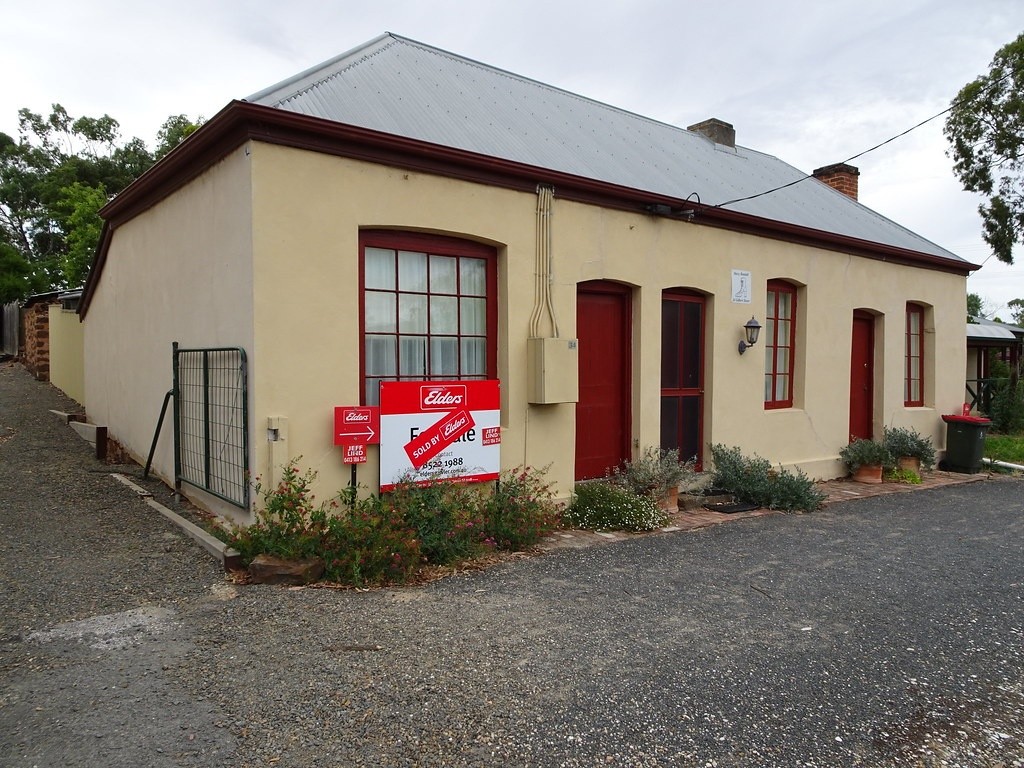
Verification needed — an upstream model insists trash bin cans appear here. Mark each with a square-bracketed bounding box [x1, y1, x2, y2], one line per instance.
[938, 414, 989, 474]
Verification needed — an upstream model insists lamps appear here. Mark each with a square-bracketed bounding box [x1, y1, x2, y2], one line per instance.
[677, 209, 694, 221]
[738, 314, 762, 355]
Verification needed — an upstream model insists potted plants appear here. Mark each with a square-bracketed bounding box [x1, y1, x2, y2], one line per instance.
[883, 425, 936, 473]
[836, 435, 891, 484]
[615, 445, 702, 513]
